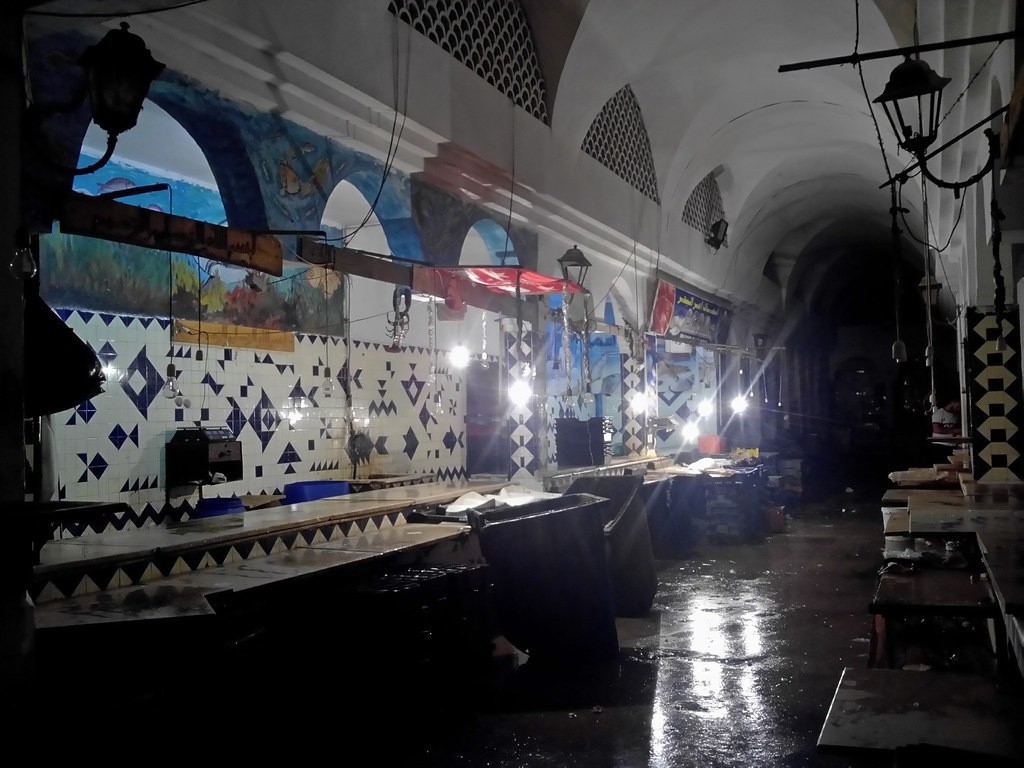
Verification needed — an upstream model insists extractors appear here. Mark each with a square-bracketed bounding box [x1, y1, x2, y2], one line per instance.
[0, 279, 107, 421]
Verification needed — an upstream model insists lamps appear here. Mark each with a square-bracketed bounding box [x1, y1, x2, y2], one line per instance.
[872, 54, 999, 196]
[891, 289, 907, 364]
[706, 220, 729, 248]
[919, 274, 941, 305]
[549, 245, 591, 316]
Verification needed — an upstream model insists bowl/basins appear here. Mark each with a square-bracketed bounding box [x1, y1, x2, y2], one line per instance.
[947, 456, 972, 464]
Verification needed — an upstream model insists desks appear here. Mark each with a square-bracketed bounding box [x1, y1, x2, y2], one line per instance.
[814, 665, 1024, 768]
[879, 489, 963, 502]
[868, 558, 1000, 667]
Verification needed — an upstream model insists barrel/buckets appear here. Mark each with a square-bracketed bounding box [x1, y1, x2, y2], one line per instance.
[882, 507, 910, 542]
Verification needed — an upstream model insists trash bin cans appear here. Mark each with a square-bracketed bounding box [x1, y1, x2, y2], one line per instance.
[466, 491, 621, 685]
[562, 472, 659, 617]
